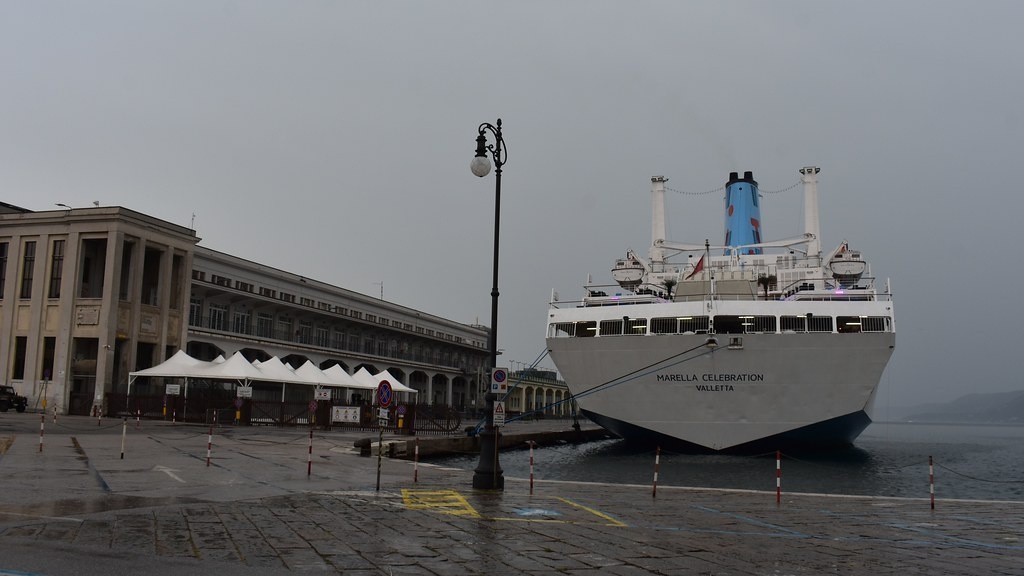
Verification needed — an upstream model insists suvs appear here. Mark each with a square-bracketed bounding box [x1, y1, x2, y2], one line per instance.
[0, 384, 28, 413]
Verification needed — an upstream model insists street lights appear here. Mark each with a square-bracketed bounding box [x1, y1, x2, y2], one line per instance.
[469, 117, 509, 492]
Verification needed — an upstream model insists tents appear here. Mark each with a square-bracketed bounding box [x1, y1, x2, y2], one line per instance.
[127, 350, 418, 429]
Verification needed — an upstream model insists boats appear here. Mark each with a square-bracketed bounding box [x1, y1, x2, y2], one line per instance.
[544, 164, 896, 457]
[829, 249, 866, 287]
[611, 258, 645, 292]
